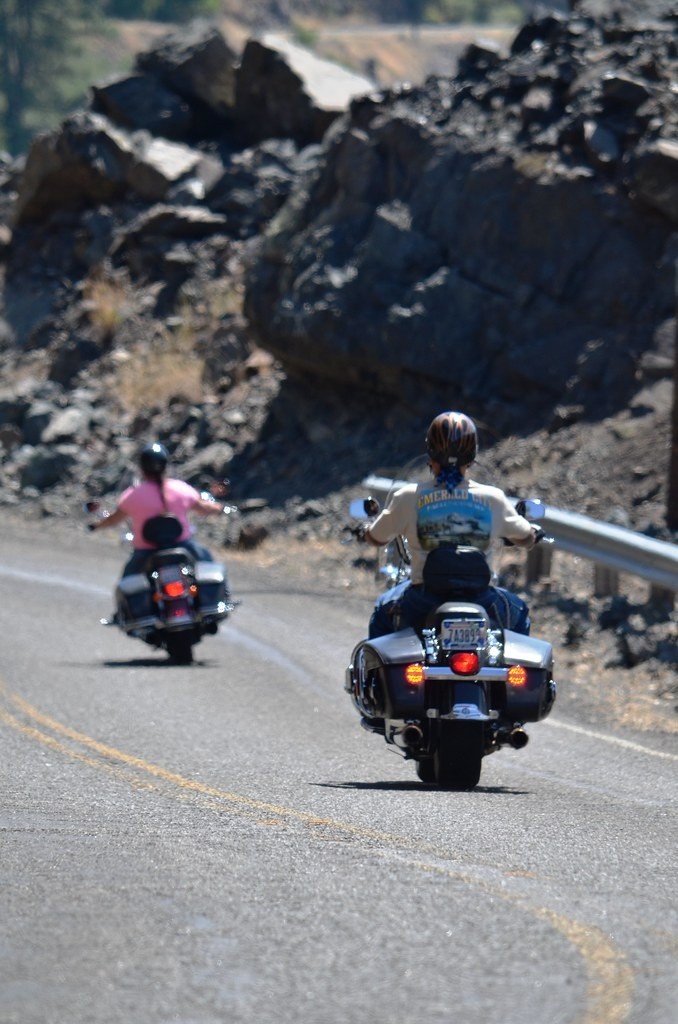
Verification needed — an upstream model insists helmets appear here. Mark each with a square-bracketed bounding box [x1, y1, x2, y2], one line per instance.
[138, 442, 168, 477]
[425, 411, 478, 467]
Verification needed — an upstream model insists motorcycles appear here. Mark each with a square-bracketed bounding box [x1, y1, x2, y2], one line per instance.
[344, 496, 559, 787]
[82, 480, 244, 664]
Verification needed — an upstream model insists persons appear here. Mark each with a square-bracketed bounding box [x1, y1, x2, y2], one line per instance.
[360, 411, 545, 733]
[93, 442, 223, 626]
